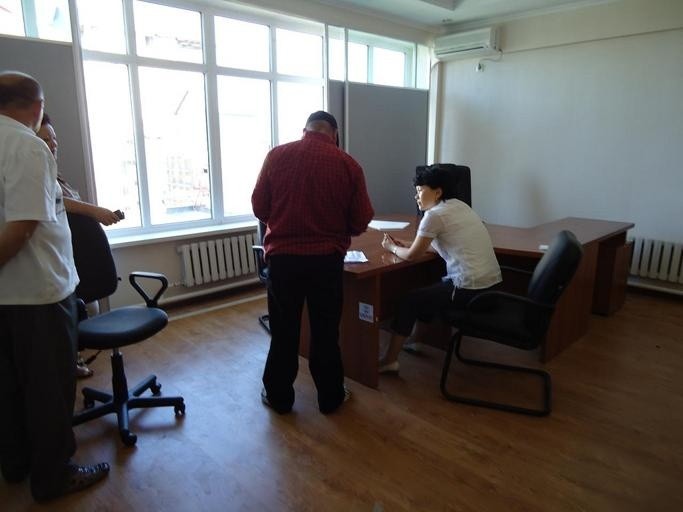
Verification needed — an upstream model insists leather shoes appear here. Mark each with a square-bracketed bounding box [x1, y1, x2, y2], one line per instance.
[401, 339, 423, 355]
[33, 462, 110, 505]
[378, 356, 399, 378]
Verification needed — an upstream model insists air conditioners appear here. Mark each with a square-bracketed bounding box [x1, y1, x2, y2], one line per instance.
[433, 26, 499, 60]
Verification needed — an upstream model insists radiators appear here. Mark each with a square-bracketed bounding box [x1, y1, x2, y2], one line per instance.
[631, 236, 683, 284]
[177, 233, 269, 288]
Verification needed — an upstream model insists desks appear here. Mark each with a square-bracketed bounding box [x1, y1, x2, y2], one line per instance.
[294, 213, 635, 392]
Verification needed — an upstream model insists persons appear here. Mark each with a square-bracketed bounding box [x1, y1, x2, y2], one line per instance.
[35, 112, 121, 375]
[251, 110, 374, 414]
[379, 168, 502, 376]
[0, 70, 108, 503]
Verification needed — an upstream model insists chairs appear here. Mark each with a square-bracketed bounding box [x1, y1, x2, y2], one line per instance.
[439, 229, 583, 416]
[69, 214, 185, 446]
[251, 220, 272, 333]
[415, 163, 472, 214]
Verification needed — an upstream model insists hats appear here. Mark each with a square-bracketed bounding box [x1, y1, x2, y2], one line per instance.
[305, 111, 338, 148]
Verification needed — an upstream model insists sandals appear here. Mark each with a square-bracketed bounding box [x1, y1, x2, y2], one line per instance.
[75, 364, 94, 381]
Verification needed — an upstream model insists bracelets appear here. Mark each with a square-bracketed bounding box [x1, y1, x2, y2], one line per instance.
[392, 245, 398, 254]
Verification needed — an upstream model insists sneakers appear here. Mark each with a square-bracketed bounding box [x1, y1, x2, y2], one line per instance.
[260, 387, 271, 407]
[342, 383, 351, 402]
